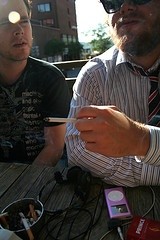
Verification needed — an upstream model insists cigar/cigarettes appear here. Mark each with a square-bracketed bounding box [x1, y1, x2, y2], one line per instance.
[0, 197, 38, 240]
[43, 117, 77, 122]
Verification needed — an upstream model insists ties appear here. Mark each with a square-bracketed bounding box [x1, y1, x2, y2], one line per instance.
[124, 62, 160, 128]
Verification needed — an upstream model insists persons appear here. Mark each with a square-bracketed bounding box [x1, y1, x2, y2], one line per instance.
[0, 0, 72, 167]
[63, 0, 160, 187]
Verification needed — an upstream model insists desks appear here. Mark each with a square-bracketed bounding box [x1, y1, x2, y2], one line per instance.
[0, 161, 160, 240]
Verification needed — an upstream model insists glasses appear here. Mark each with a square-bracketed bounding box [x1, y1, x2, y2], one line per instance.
[100, 0, 150, 14]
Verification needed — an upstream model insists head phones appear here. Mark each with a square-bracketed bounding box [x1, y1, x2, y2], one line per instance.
[37, 166, 91, 214]
